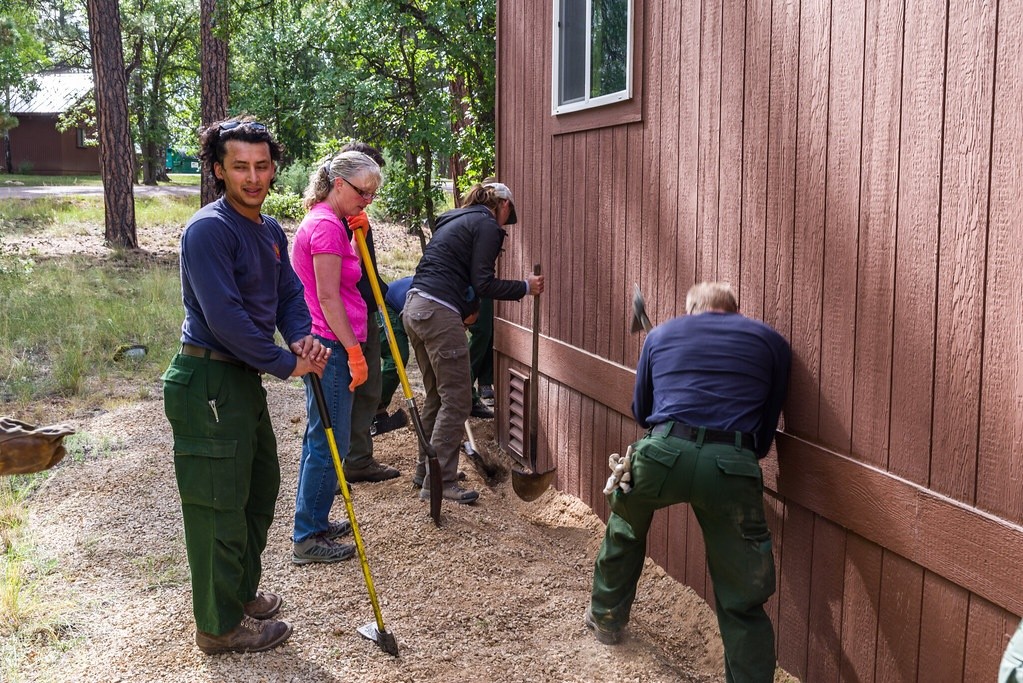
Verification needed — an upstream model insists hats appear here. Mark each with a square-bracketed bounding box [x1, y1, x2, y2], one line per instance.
[483, 182, 517, 225]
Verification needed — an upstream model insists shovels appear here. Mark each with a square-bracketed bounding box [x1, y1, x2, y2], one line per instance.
[511, 264, 558, 503]
[352, 227, 444, 527]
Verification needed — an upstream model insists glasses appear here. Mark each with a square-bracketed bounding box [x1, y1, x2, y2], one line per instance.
[217, 121, 270, 153]
[333, 177, 377, 200]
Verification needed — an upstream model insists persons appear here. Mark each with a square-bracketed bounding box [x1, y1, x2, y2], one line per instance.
[291, 149, 401, 564]
[372, 183, 545, 503]
[583, 282, 791, 683]
[160, 113, 333, 655]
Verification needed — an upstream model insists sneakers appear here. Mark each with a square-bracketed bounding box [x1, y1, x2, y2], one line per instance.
[291, 532, 356, 563]
[244, 591, 282, 620]
[419, 487, 479, 504]
[412, 469, 466, 487]
[309, 519, 351, 540]
[195, 615, 293, 656]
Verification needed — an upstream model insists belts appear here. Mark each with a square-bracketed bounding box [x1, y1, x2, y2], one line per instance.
[179, 343, 235, 362]
[652, 422, 760, 452]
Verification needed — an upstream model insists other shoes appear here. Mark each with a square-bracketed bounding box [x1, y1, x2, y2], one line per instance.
[585, 606, 622, 645]
[343, 459, 400, 484]
[333, 480, 351, 495]
[478, 382, 495, 406]
[470, 399, 494, 418]
[372, 411, 389, 425]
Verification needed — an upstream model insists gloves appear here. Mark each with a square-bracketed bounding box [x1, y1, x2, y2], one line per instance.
[346, 343, 368, 392]
[345, 210, 369, 241]
[602, 444, 633, 494]
